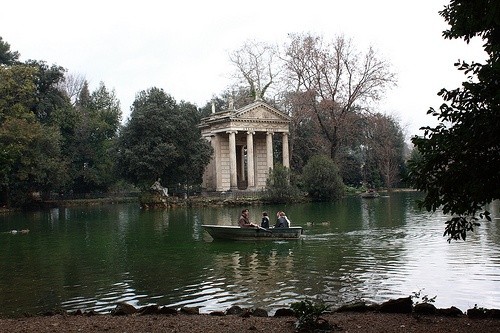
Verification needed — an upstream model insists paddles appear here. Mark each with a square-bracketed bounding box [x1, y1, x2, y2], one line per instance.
[250, 222, 271, 233]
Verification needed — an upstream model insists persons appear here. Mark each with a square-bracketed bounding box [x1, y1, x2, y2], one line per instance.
[273, 210, 290, 228]
[261, 211, 270, 229]
[238, 208, 258, 229]
[150, 176, 169, 197]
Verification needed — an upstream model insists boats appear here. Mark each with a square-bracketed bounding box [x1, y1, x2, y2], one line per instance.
[200, 223, 303, 240]
[361, 190, 379, 198]
